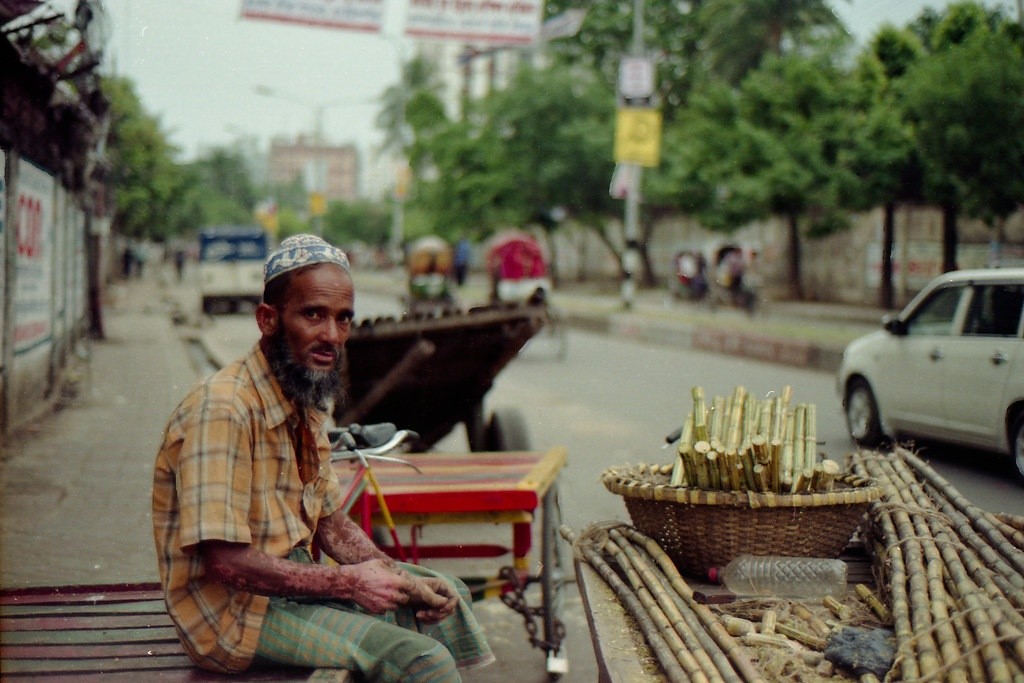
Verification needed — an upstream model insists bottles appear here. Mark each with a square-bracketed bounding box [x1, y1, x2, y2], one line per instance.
[707, 556, 850, 598]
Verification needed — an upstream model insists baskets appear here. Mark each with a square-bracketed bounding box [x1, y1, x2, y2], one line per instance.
[600, 461, 881, 585]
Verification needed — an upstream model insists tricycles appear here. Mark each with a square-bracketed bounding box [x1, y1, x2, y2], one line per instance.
[323, 423, 579, 683]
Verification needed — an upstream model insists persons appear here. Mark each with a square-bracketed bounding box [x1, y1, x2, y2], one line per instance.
[122, 241, 146, 274]
[455, 234, 470, 286]
[621, 240, 648, 308]
[717, 247, 756, 309]
[175, 245, 183, 273]
[152, 233, 496, 682]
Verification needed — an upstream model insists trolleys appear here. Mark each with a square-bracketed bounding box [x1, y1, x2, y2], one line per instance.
[326, 281, 548, 452]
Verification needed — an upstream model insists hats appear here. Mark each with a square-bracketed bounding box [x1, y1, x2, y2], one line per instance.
[264, 234, 349, 288]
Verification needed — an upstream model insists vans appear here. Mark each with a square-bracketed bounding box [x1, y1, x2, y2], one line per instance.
[199, 229, 270, 316]
[834, 265, 1024, 488]
